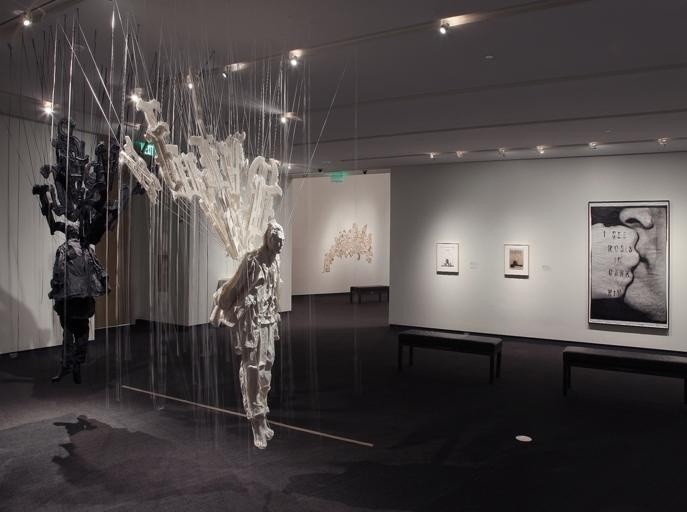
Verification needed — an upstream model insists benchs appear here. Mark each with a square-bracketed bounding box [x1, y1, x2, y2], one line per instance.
[351, 286, 389, 304]
[397, 329, 503, 386]
[562, 346, 687, 404]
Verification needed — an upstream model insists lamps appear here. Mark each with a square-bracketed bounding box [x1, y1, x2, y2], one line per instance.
[23, 15, 33, 26]
[221, 71, 229, 78]
[439, 23, 449, 34]
[291, 56, 297, 65]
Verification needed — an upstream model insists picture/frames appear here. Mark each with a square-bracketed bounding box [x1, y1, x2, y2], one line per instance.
[436, 242, 458, 273]
[504, 244, 529, 276]
[588, 200, 670, 329]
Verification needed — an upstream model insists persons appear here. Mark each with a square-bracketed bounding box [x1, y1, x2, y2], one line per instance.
[49, 221, 111, 384]
[617, 206, 667, 325]
[589, 222, 641, 302]
[217, 222, 284, 451]
